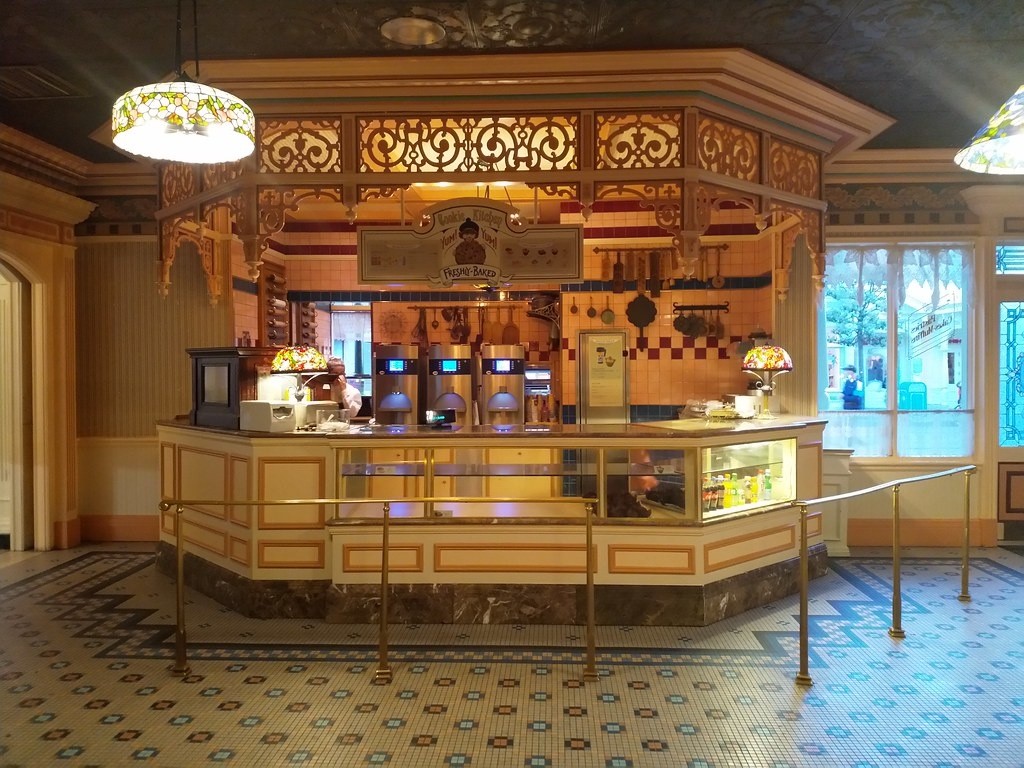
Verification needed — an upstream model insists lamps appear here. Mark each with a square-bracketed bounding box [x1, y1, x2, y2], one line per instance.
[110, 0, 256, 163]
[953, 84, 1024, 176]
[269, 342, 330, 429]
[739, 346, 793, 422]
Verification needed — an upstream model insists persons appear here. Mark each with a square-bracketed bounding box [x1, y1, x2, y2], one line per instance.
[326, 356, 362, 418]
[839, 365, 864, 409]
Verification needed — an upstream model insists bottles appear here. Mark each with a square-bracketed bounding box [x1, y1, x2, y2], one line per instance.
[266, 274, 286, 284]
[268, 298, 287, 308]
[531, 399, 550, 423]
[702, 469, 772, 512]
[267, 320, 288, 327]
[301, 301, 319, 348]
[268, 287, 286, 295]
[268, 332, 289, 340]
[241, 331, 251, 347]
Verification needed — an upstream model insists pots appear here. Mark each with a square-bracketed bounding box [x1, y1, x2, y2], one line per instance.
[570, 297, 578, 314]
[600, 296, 615, 324]
[587, 296, 596, 317]
